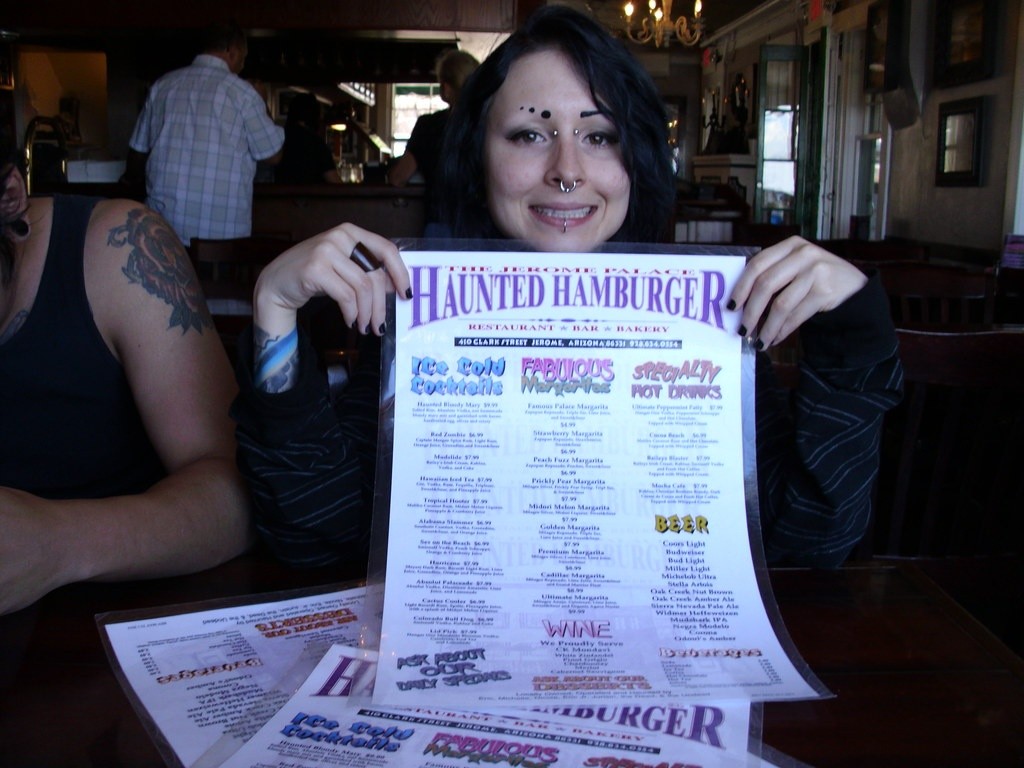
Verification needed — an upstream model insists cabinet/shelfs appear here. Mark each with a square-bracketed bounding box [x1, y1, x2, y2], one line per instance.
[692, 154, 756, 208]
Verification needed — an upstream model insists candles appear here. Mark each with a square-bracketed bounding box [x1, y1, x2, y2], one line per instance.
[700, 76, 750, 116]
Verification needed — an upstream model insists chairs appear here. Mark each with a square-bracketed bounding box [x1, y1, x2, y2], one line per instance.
[189, 234, 293, 296]
[840, 255, 999, 328]
[843, 322, 1023, 656]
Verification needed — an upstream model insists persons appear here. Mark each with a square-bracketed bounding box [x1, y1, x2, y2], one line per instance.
[388, 48, 484, 239]
[232, 2, 903, 575]
[276, 91, 339, 183]
[1, 121, 250, 617]
[125, 11, 288, 305]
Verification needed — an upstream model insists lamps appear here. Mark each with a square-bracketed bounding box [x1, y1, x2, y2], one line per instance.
[620, 0, 706, 48]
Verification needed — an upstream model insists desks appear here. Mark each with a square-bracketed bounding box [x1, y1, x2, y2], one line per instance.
[0, 550, 1024, 768]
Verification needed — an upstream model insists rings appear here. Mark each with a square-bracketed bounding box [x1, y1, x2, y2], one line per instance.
[349, 241, 381, 272]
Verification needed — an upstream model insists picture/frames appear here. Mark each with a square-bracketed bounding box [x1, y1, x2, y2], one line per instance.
[924, 1, 1003, 92]
[863, 0, 906, 96]
[935, 94, 993, 189]
